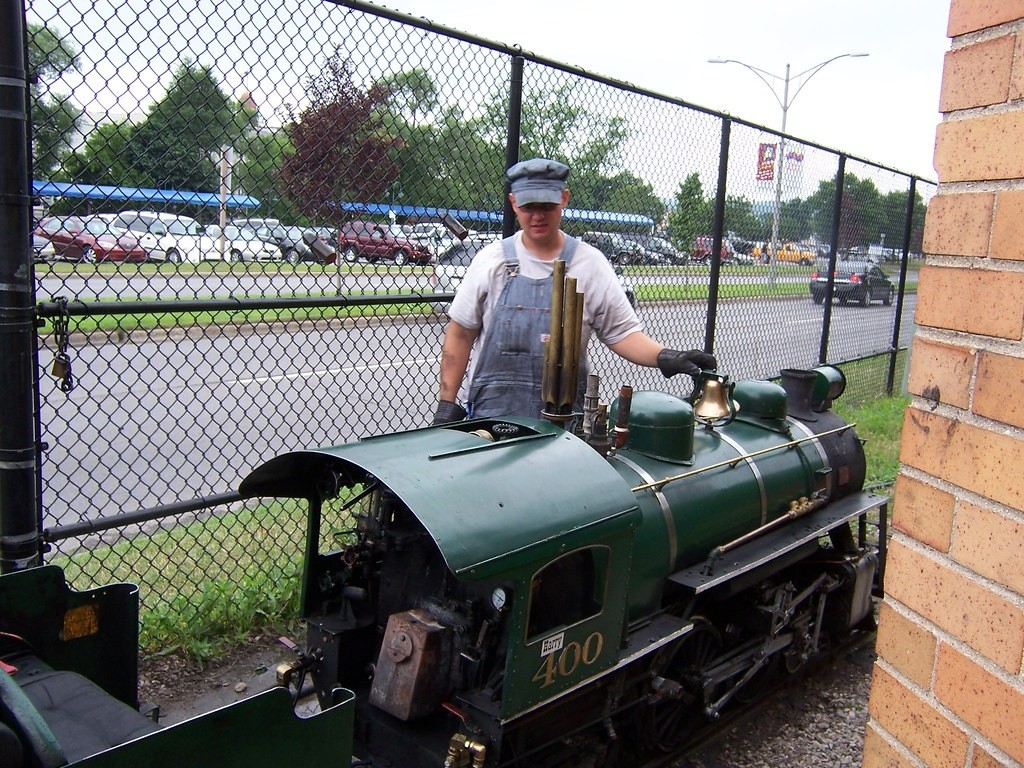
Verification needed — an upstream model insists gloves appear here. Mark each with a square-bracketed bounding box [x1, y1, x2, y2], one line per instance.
[658, 348, 717, 379]
[431, 399, 468, 426]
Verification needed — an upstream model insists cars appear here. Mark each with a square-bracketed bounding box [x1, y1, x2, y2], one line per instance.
[806, 259, 896, 308]
[28, 209, 912, 278]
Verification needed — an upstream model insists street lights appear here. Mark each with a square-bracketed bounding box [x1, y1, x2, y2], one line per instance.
[708, 49, 869, 287]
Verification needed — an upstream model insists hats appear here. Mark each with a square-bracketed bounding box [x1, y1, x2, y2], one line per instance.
[505, 158, 570, 207]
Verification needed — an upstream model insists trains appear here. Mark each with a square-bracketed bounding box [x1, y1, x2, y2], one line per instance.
[0, 257, 897, 768]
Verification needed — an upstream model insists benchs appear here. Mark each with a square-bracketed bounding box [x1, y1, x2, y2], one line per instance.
[0, 648, 167, 768]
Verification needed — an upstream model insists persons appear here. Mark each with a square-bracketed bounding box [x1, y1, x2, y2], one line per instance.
[760, 239, 782, 264]
[432, 158, 718, 426]
